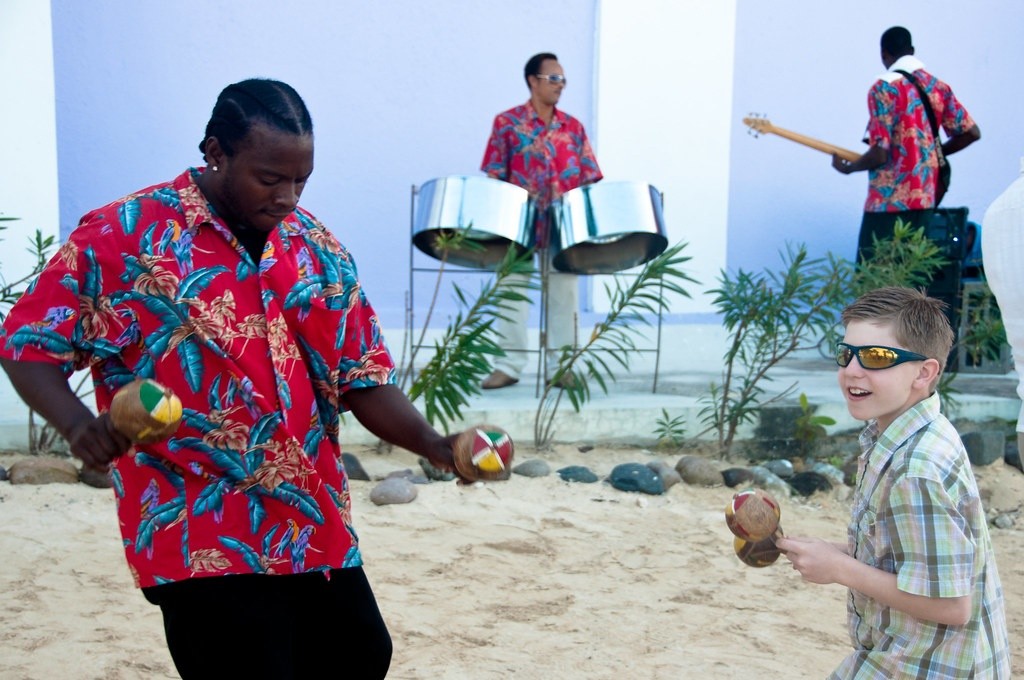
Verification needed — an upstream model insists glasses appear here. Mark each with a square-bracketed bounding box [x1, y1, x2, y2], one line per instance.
[537, 74, 566, 84]
[837, 343, 928, 370]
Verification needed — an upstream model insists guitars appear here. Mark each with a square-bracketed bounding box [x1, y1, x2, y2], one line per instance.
[742, 112, 951, 208]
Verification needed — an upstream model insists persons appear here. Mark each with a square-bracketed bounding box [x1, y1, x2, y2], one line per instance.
[775, 286, 1011, 680]
[480, 53, 604, 390]
[0, 78, 515, 680]
[833, 26, 980, 283]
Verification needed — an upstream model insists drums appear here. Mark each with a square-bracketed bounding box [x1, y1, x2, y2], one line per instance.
[410, 174, 541, 273]
[542, 179, 669, 276]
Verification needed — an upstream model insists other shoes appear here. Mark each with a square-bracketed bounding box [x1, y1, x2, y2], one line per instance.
[482, 368, 519, 388]
[547, 372, 584, 390]
